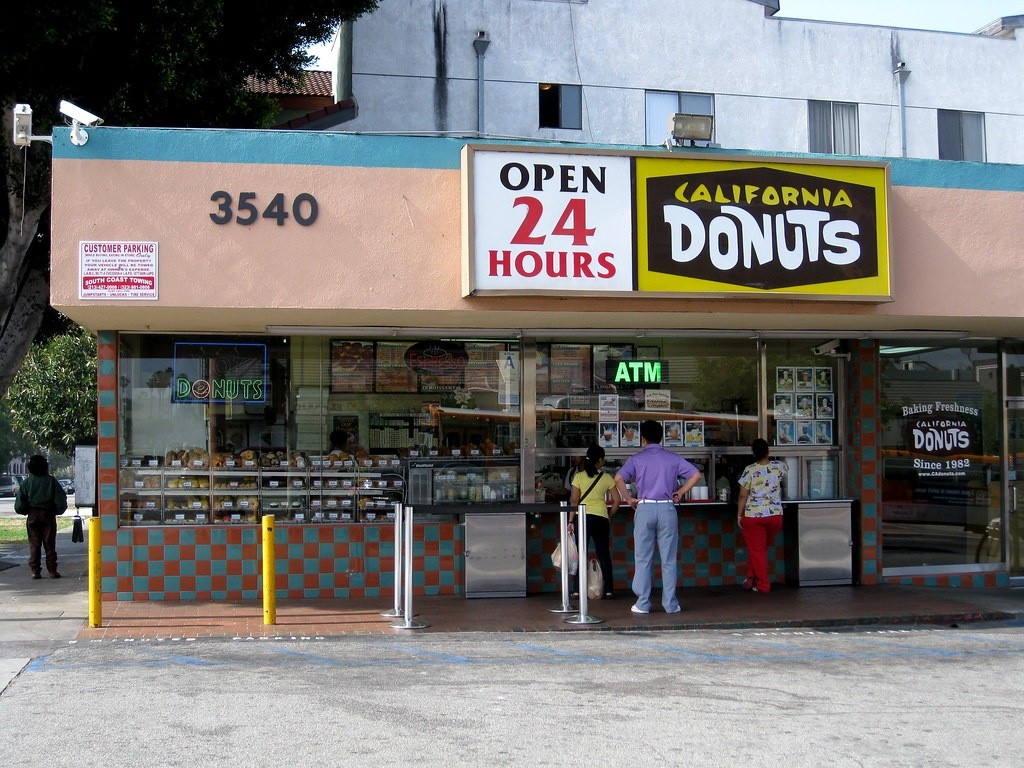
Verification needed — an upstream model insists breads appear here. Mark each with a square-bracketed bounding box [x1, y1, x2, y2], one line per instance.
[165, 447, 209, 470]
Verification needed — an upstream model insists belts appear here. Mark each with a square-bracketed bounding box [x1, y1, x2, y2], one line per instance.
[637, 499, 674, 503]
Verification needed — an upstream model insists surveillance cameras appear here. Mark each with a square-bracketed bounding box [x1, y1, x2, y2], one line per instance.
[811, 339, 840, 355]
[14, 104, 32, 147]
[59, 100, 104, 129]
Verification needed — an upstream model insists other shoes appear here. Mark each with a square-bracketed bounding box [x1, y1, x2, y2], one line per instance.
[674, 605, 680, 613]
[606, 593, 615, 600]
[571, 593, 578, 599]
[743, 576, 759, 592]
[631, 605, 649, 614]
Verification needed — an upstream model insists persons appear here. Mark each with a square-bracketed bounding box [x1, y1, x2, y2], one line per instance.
[14, 455, 69, 580]
[737, 438, 789, 593]
[614, 419, 703, 614]
[567, 444, 621, 600]
[564, 456, 586, 492]
[329, 429, 348, 457]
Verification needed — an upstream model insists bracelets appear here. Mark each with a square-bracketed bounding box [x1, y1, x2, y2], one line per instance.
[737, 517, 743, 519]
[569, 521, 574, 523]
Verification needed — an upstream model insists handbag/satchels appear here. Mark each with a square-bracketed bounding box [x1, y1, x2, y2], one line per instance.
[588, 559, 603, 599]
[551, 531, 578, 575]
